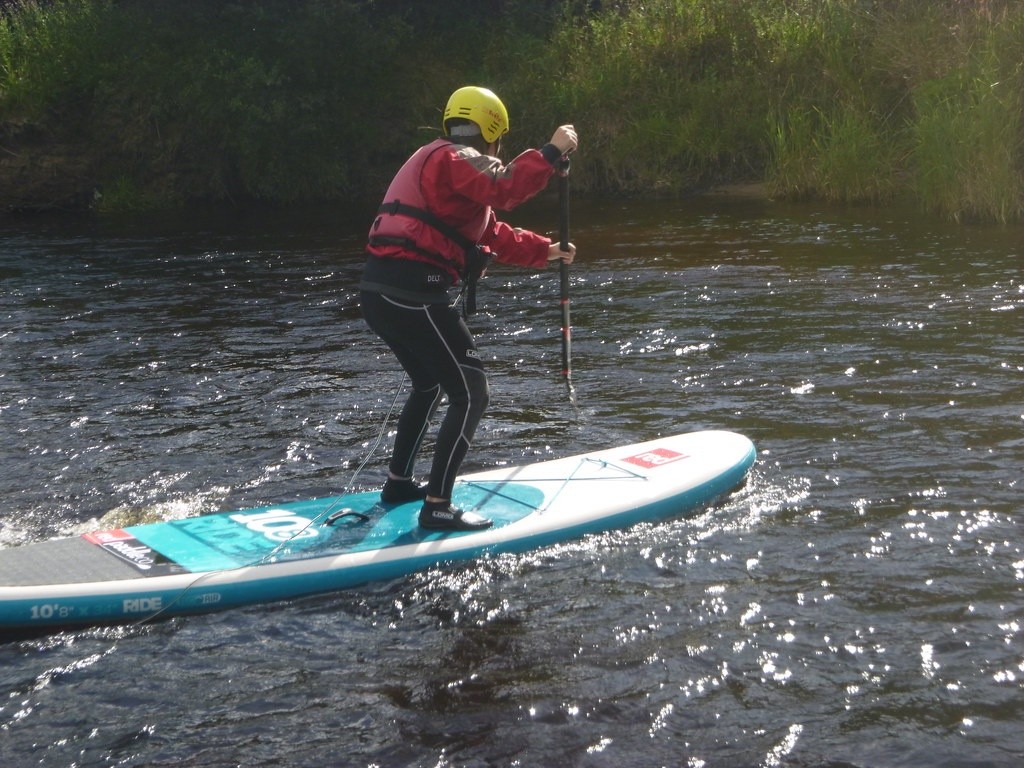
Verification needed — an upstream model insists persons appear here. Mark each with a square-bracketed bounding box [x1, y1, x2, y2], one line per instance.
[359, 85, 578, 530]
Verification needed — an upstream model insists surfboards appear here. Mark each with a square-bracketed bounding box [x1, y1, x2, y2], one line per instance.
[0, 429, 760, 629]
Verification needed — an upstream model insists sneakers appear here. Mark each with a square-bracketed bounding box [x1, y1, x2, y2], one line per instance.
[381, 475, 427, 502]
[419, 499, 493, 531]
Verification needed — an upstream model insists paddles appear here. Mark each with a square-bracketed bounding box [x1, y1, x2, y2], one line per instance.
[562, 150, 577, 399]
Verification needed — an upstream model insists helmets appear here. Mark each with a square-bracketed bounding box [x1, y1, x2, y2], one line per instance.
[441, 86, 509, 143]
